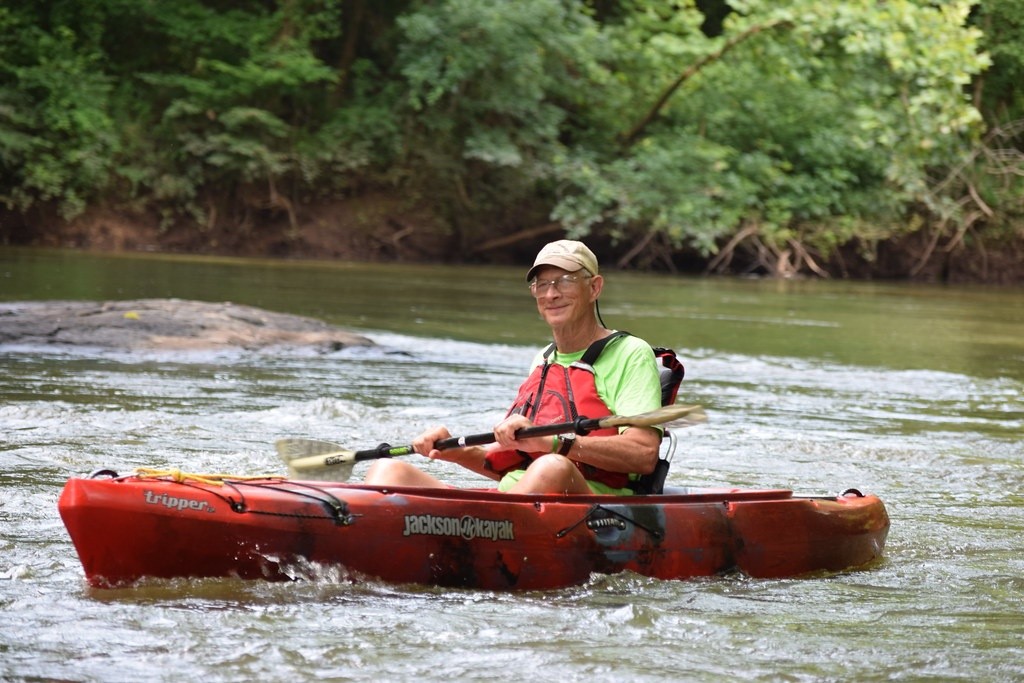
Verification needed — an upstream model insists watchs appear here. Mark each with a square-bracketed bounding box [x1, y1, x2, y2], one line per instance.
[558, 432, 576, 456]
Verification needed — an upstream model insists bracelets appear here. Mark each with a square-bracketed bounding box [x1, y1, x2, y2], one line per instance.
[551, 434, 559, 454]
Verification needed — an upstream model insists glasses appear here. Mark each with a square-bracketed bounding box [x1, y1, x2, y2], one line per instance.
[527, 274, 590, 297]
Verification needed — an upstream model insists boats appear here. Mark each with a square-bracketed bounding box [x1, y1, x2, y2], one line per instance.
[57, 467, 891, 595]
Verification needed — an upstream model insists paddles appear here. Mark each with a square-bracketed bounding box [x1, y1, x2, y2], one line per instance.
[270, 404, 703, 483]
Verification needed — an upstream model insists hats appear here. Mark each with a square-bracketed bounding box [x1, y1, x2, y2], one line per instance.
[526, 240, 599, 282]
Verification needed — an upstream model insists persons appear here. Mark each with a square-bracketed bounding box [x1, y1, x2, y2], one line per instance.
[363, 240, 663, 495]
